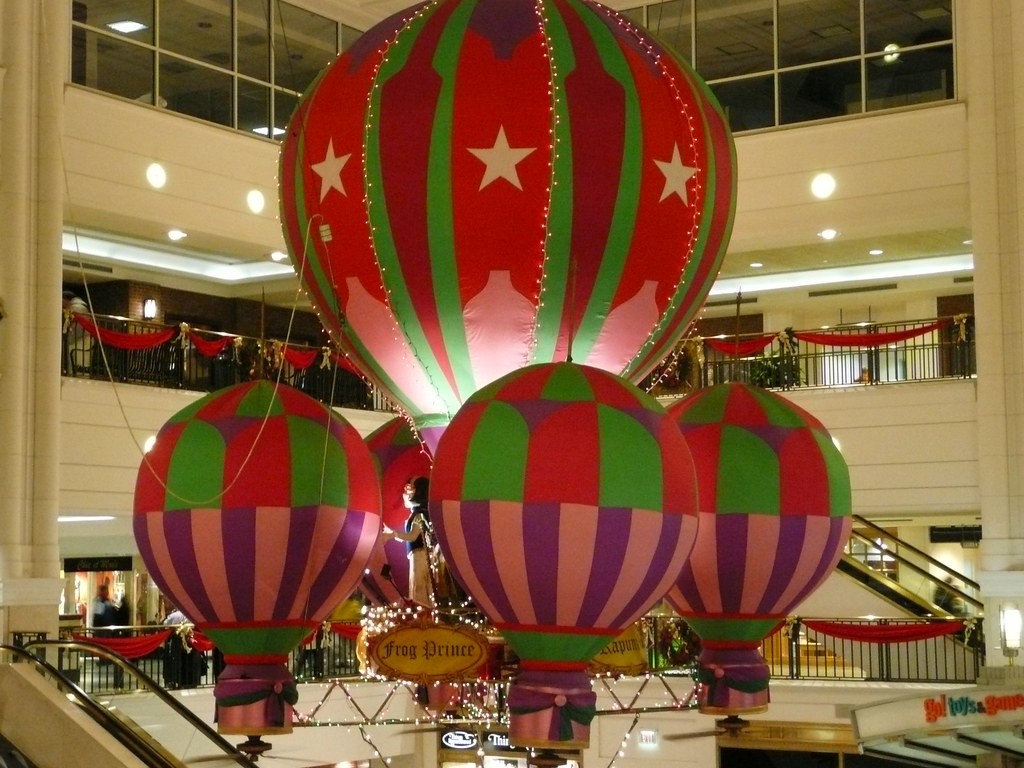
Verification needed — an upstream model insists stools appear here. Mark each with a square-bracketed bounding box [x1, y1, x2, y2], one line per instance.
[10, 630, 51, 678]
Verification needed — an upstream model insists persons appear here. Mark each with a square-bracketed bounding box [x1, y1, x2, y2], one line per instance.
[117, 597, 130, 626]
[92, 585, 115, 614]
[380, 477, 436, 607]
[855, 366, 869, 382]
[932, 575, 970, 618]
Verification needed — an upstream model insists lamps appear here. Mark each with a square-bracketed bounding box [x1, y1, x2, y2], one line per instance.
[144, 297, 157, 319]
[999, 600, 1022, 667]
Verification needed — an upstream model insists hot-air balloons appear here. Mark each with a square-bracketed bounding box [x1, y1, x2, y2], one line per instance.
[276, 1, 743, 461]
[657, 377, 855, 737]
[129, 377, 384, 765]
[357, 414, 502, 681]
[424, 357, 699, 768]
